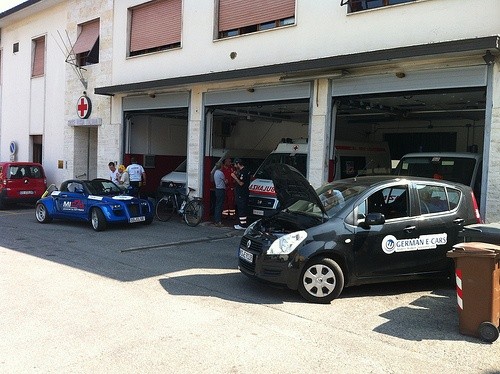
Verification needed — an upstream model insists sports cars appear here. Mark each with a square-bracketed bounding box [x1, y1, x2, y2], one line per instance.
[34, 178, 158, 231]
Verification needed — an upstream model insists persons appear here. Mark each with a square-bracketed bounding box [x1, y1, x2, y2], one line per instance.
[313, 182, 348, 220]
[213, 158, 248, 230]
[108, 156, 146, 199]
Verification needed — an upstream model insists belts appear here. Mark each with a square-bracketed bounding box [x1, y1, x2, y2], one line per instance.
[217, 189, 226, 190]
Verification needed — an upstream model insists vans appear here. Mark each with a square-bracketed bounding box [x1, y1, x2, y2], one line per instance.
[0, 162, 51, 207]
[387, 151, 483, 216]
[159, 146, 270, 215]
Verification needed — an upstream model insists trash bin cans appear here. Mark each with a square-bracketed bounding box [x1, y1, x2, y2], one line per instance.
[460, 223, 500, 242]
[446, 242, 500, 344]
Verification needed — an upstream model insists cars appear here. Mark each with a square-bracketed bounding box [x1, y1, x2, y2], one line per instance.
[237, 163, 484, 304]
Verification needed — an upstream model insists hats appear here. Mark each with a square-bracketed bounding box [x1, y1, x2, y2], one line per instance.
[115, 164, 125, 170]
[232, 159, 241, 164]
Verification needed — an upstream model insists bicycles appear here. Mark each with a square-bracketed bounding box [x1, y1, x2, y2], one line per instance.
[155, 180, 207, 228]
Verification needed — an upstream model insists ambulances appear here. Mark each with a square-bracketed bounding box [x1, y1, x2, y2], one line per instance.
[246, 142, 340, 226]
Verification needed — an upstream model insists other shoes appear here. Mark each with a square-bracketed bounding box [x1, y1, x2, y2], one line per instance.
[233, 224, 246, 229]
[216, 222, 224, 227]
